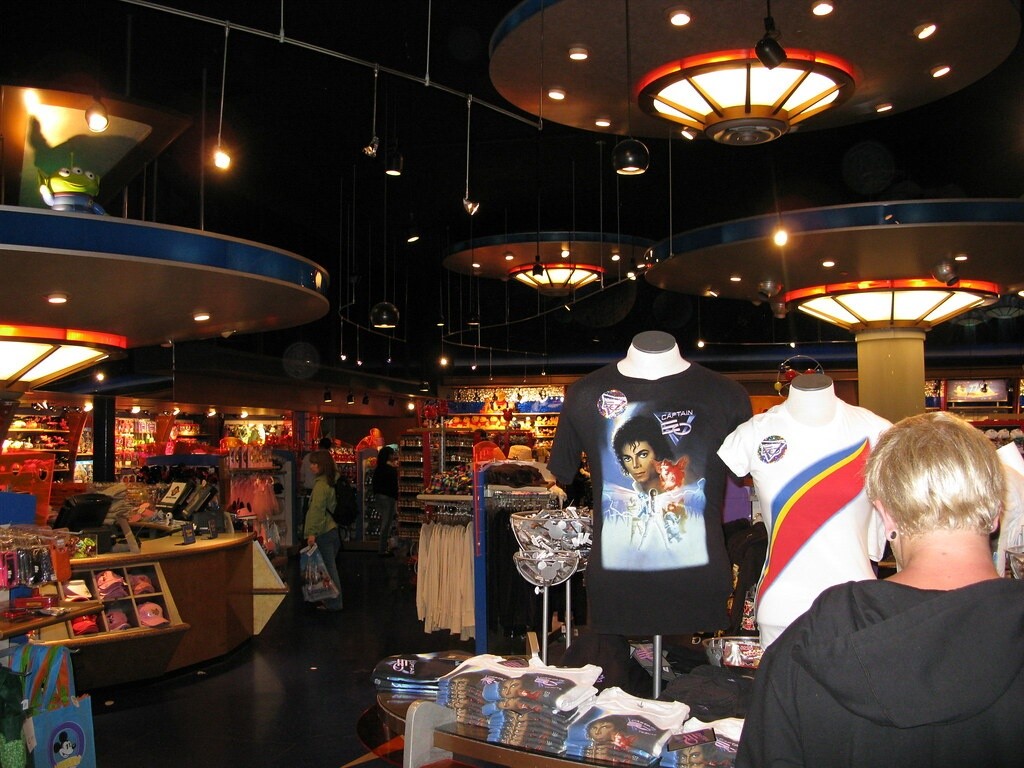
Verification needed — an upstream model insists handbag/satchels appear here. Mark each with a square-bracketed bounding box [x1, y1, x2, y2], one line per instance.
[10, 643, 100, 768]
[299, 543, 340, 609]
[385, 522, 399, 554]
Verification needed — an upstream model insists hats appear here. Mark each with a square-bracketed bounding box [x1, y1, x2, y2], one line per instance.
[60, 568, 174, 639]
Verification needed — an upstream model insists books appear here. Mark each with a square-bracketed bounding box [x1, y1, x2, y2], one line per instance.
[155, 481, 193, 508]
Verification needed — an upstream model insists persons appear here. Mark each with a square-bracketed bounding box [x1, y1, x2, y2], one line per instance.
[474, 429, 486, 441]
[732, 411, 1024, 768]
[612, 415, 674, 496]
[716, 371, 900, 656]
[372, 446, 399, 559]
[304, 451, 343, 611]
[300, 438, 337, 541]
[545, 328, 754, 638]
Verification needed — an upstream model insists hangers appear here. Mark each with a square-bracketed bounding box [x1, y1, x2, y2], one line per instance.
[423, 490, 558, 530]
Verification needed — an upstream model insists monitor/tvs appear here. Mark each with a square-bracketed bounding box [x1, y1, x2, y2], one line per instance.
[154, 481, 196, 514]
[53, 494, 114, 533]
[181, 484, 218, 519]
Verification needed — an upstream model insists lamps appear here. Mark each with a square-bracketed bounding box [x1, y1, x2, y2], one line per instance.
[750, 2, 793, 70]
[339, 68, 421, 365]
[163, 407, 180, 417]
[608, 138, 657, 178]
[323, 390, 333, 403]
[0, 319, 126, 401]
[505, 262, 605, 298]
[784, 276, 1001, 336]
[632, 48, 860, 146]
[205, 407, 216, 416]
[205, 27, 241, 177]
[418, 379, 432, 392]
[346, 390, 356, 405]
[459, 93, 482, 218]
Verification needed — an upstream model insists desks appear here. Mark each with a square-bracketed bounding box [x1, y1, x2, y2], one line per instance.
[0, 601, 104, 659]
[376, 654, 636, 768]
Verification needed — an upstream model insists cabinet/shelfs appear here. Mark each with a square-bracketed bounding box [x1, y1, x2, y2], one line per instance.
[31, 561, 193, 648]
[332, 446, 382, 542]
[396, 428, 530, 541]
[535, 425, 556, 450]
[0, 401, 294, 559]
